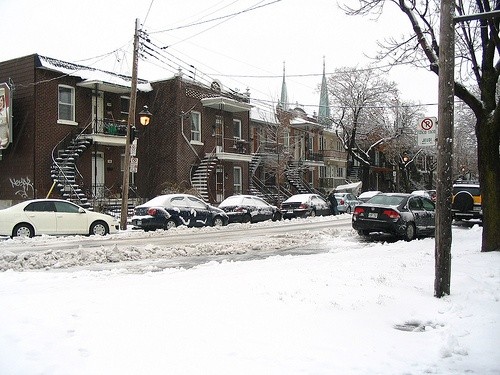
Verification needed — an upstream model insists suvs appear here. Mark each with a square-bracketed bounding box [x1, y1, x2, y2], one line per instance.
[452, 184, 483, 223]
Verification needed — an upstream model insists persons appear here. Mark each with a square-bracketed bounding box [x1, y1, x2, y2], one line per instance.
[327, 194, 338, 216]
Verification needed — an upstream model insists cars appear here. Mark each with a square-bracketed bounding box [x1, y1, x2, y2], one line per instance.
[411, 189, 437, 202]
[132, 194, 229, 232]
[333, 192, 358, 214]
[359, 191, 383, 204]
[0, 199, 120, 239]
[281, 194, 330, 220]
[217, 194, 280, 224]
[352, 193, 436, 242]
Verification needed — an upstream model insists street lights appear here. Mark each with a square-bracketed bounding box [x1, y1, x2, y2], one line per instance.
[396, 151, 408, 192]
[121, 105, 152, 230]
